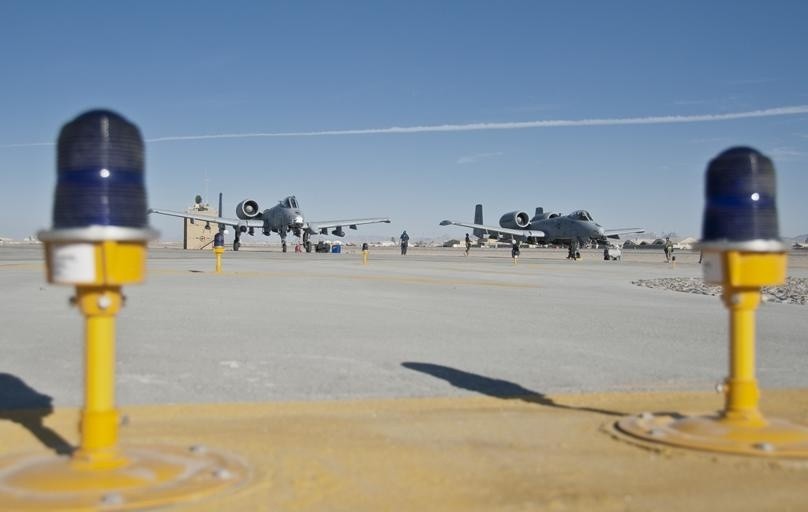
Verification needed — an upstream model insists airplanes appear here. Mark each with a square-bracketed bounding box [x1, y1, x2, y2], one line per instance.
[148, 193, 390, 252]
[440, 204, 648, 259]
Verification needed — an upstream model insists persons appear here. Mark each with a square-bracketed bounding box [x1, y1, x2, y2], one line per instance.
[664, 237, 672, 262]
[566, 241, 577, 260]
[400, 230, 410, 256]
[464, 233, 473, 256]
[697, 249, 703, 264]
[603, 241, 613, 260]
[511, 239, 520, 264]
